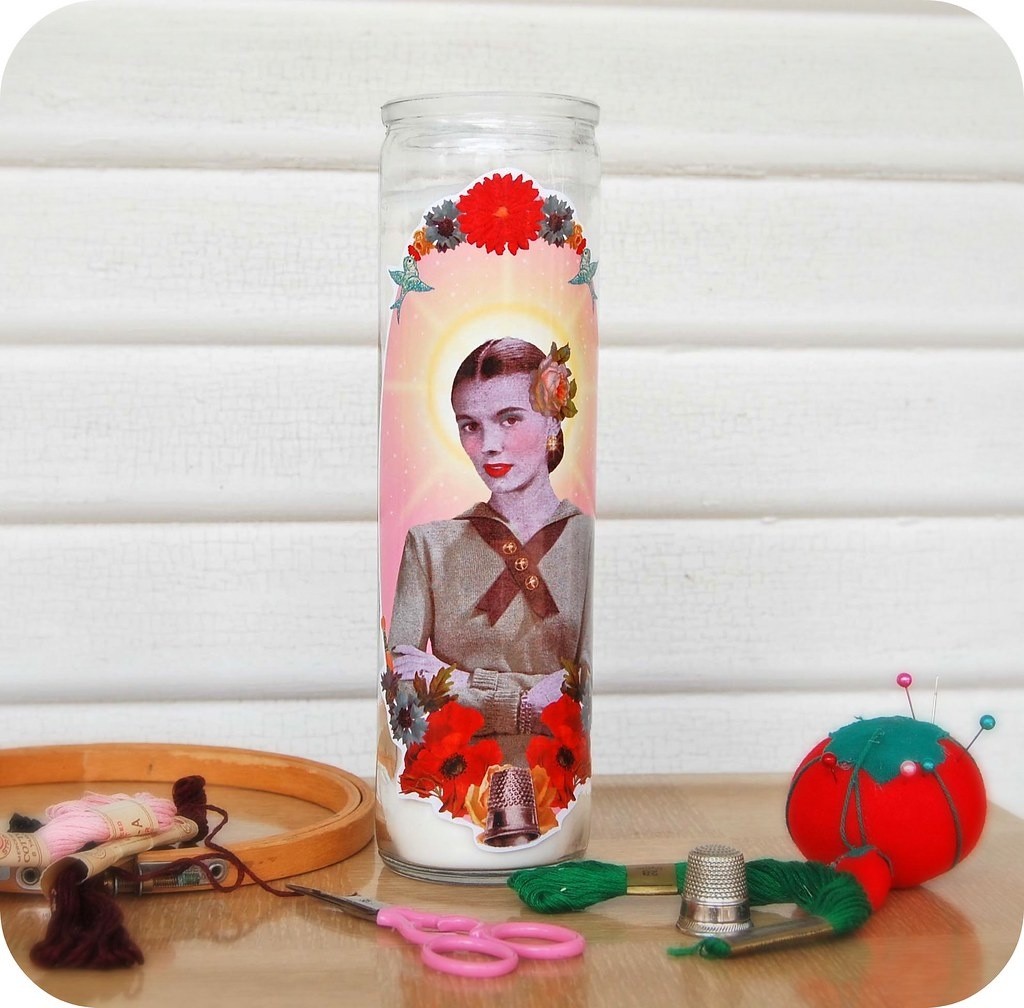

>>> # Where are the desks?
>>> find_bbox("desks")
[0,768,1024,1008]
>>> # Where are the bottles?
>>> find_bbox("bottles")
[367,93,600,884]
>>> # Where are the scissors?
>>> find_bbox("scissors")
[285,883,586,980]
